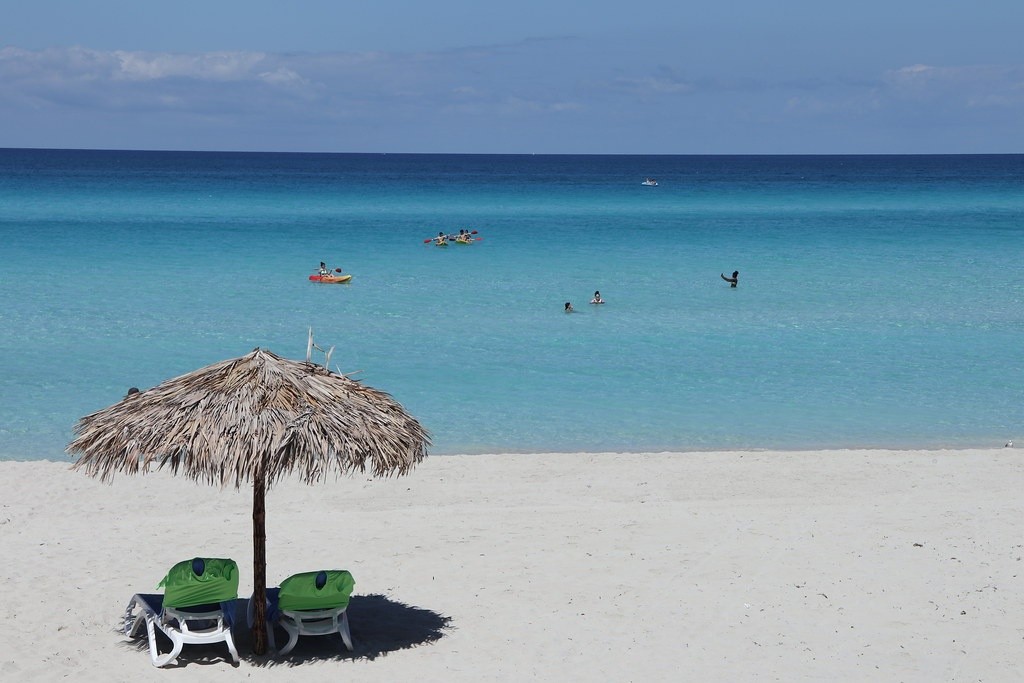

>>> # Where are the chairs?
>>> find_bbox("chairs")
[248,570,355,657]
[125,558,240,669]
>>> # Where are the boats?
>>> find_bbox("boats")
[309,270,352,283]
[641,181,659,186]
[436,241,448,247]
[456,238,472,244]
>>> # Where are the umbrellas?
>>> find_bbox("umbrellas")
[64,325,435,653]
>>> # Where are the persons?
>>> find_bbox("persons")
[591,291,605,303]
[433,232,448,243]
[565,302,573,312]
[456,230,471,242]
[650,179,656,184]
[721,271,739,288]
[318,262,334,277]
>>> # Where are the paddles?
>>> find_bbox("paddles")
[314,268,341,273]
[424,230,482,243]
